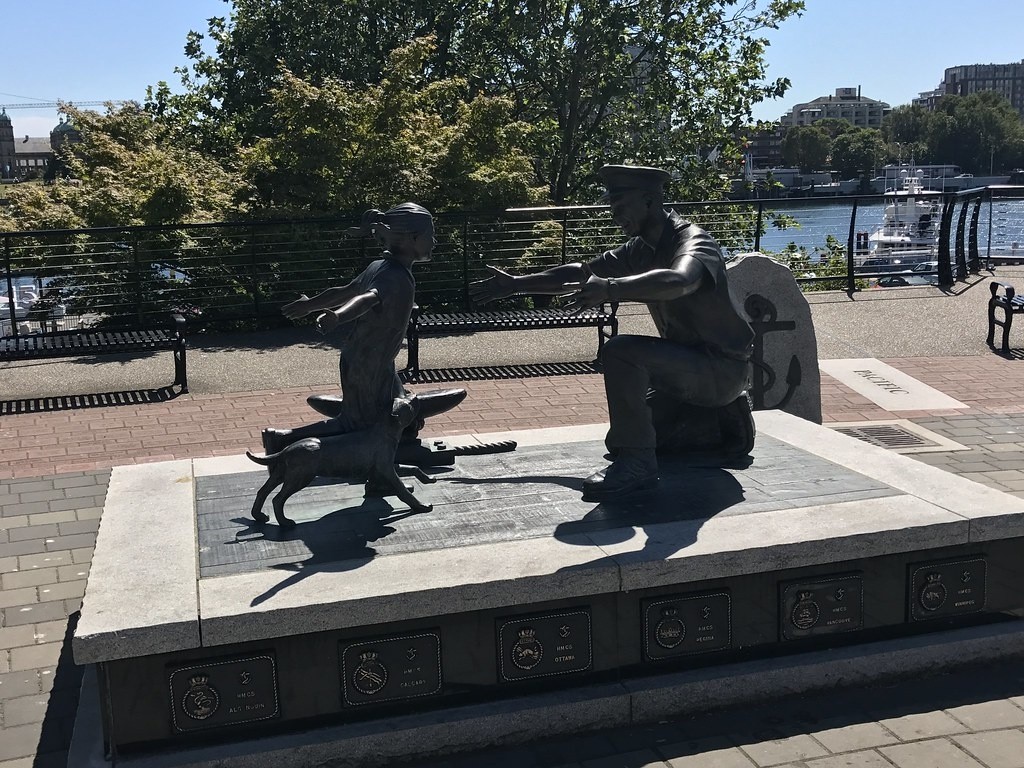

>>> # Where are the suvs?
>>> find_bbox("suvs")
[870,177,886,181]
[954,173,973,178]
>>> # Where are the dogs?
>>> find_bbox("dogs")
[245,393,434,527]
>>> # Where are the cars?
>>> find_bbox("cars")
[1012,169,1024,174]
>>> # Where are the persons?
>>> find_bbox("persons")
[468,165,756,498]
[262,202,437,479]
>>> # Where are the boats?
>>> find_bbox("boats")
[856,141,957,275]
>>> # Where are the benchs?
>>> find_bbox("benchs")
[985,281,1024,351]
[408,293,619,373]
[0,314,189,393]
